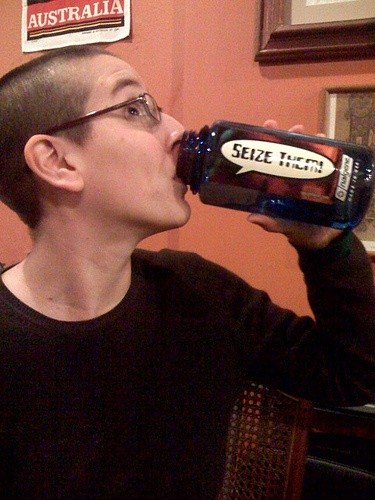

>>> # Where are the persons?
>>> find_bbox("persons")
[0,45,375,500]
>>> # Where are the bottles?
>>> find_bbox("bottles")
[174,120,375,230]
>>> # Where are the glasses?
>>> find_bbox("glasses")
[22,93,162,173]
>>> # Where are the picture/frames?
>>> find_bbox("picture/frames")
[317,83,375,260]
[253,0,375,62]
